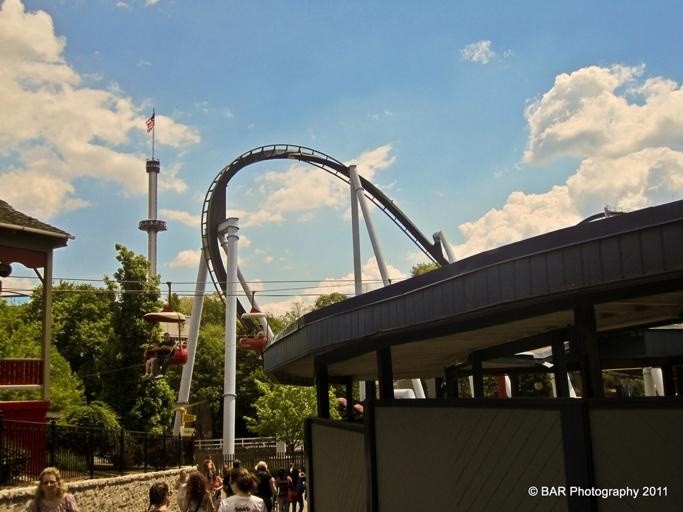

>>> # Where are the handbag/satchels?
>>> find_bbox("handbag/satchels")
[296,479,304,494]
[287,488,299,502]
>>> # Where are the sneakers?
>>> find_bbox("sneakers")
[141,373,165,380]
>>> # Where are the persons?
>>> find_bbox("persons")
[255,324,265,339]
[30,465,78,512]
[153,332,176,379]
[143,339,160,379]
[148,450,307,512]
